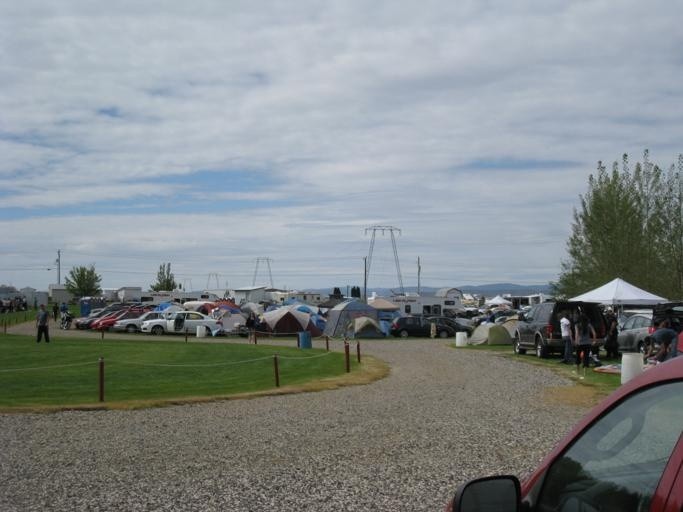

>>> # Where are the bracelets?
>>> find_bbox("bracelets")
[654,355,657,359]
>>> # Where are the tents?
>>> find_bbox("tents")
[322,299,380,337]
[151,297,326,336]
[452,311,529,345]
[339,316,389,338]
[368,298,402,328]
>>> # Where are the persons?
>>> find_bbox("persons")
[35,304,50,344]
[560,310,574,362]
[51,302,59,322]
[0,294,38,313]
[244,310,257,344]
[642,327,680,363]
[571,312,597,380]
[603,306,619,359]
[59,302,68,318]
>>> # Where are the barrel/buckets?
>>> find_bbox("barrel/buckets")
[197,326,205,337]
[298,332,312,349]
[620,353,643,383]
[456,332,467,347]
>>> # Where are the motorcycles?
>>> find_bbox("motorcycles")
[60,311,75,330]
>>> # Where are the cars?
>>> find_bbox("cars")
[74,300,223,336]
[388,310,507,338]
[447,345,683,512]
[514,299,681,358]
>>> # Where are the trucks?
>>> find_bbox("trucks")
[3,300,25,312]
